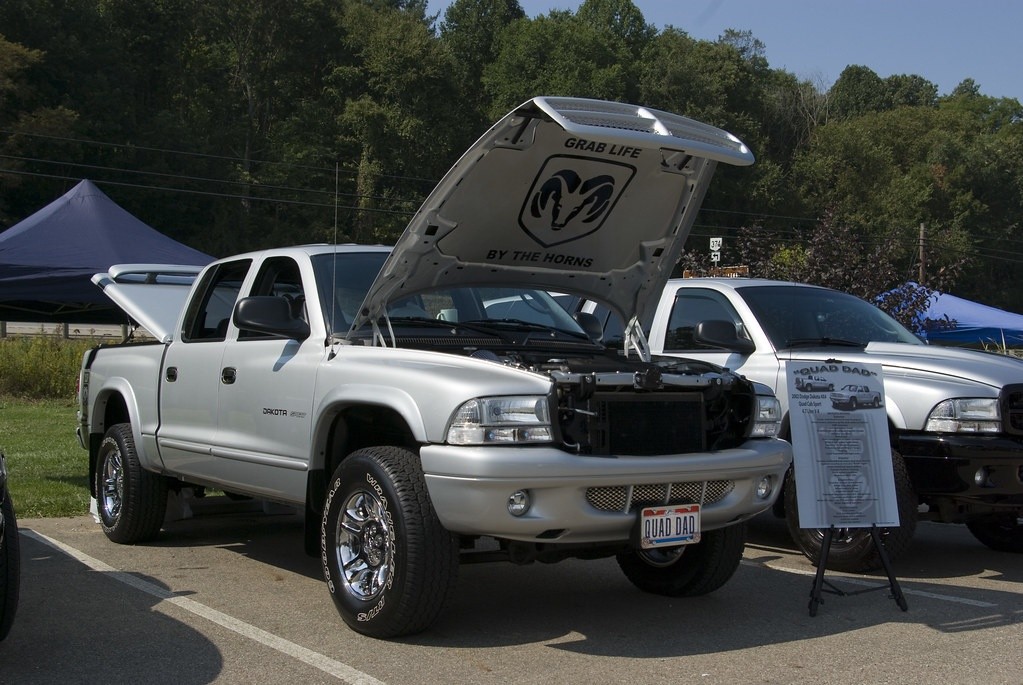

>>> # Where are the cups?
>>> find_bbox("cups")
[437,309,457,323]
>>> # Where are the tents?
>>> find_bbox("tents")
[854,281,1023,358]
[0,179,223,337]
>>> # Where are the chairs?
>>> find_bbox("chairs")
[214,317,229,338]
[289,293,345,326]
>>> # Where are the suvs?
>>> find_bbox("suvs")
[829,384,881,409]
[76,97,792,638]
[435,274,1023,571]
[795,375,835,392]
